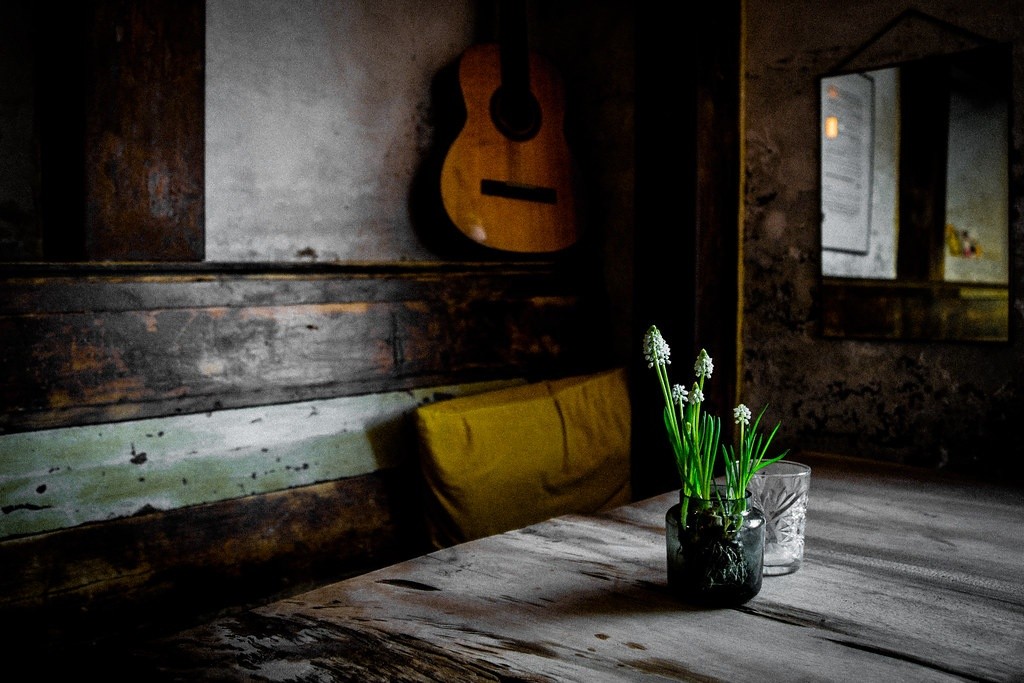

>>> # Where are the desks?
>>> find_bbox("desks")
[159,460,1024,683]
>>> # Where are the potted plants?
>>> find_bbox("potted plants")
[642,325,791,609]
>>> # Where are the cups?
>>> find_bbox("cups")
[725,459,812,576]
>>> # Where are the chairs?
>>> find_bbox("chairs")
[415,367,634,551]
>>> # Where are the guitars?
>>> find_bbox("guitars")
[423,0,578,254]
